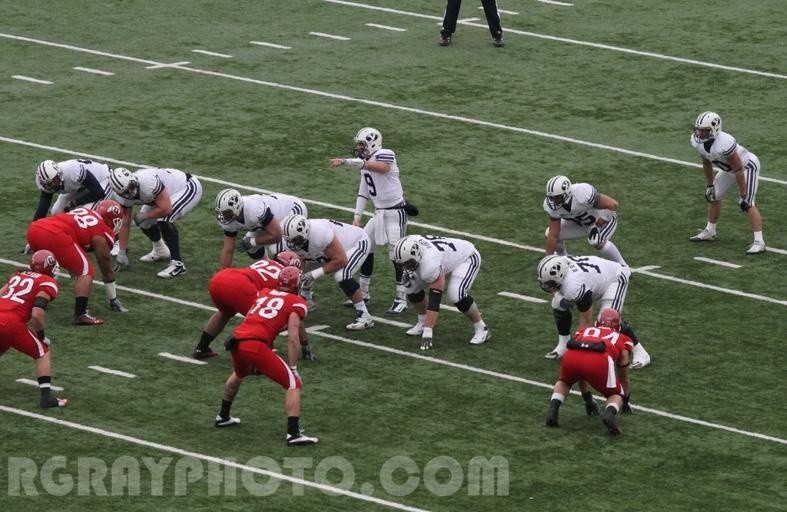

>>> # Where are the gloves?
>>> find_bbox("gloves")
[705,185,716,202]
[110,295,128,312]
[581,391,599,415]
[303,347,316,362]
[621,395,633,415]
[419,327,433,350]
[738,197,749,211]
[37,329,50,345]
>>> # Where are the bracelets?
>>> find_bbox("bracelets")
[103,279,117,301]
[249,237,256,247]
[421,326,433,338]
[310,266,325,280]
[582,391,592,401]
[36,329,45,340]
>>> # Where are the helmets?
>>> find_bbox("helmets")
[546,175,574,210]
[109,168,140,201]
[95,201,125,236]
[693,110,723,144]
[395,237,424,275]
[352,127,383,160]
[215,186,309,293]
[536,255,569,294]
[594,308,620,333]
[36,158,65,194]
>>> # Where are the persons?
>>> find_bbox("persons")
[542,175,633,281]
[328,126,408,314]
[536,254,651,370]
[281,214,375,330]
[214,265,319,446]
[191,251,318,363]
[26,199,129,325]
[21,156,115,256]
[689,111,766,254]
[438,0,505,47]
[546,308,632,435]
[109,167,203,279]
[395,234,492,351]
[0,249,69,408]
[214,188,309,271]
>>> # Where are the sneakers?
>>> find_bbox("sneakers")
[628,352,651,370]
[547,408,558,426]
[602,413,623,435]
[490,36,505,48]
[214,413,242,426]
[620,263,632,280]
[546,345,568,359]
[689,228,717,241]
[286,430,319,447]
[748,240,765,254]
[193,348,219,359]
[109,237,185,278]
[72,309,106,325]
[299,291,491,346]
[41,396,69,408]
[438,34,452,46]
